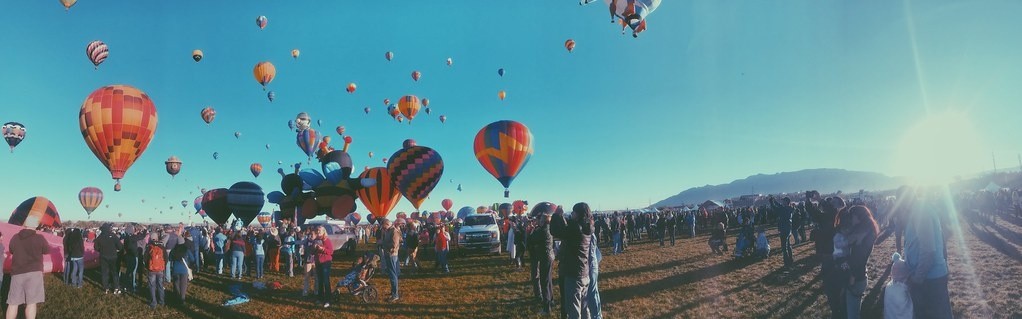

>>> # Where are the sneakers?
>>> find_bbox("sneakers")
[103,289,109,295]
[121,287,127,293]
[388,296,400,303]
[113,288,122,295]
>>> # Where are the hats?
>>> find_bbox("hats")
[150,233,159,242]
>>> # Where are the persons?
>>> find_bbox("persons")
[143,232,167,308]
[524,214,556,314]
[0,197,1021,270]
[805,190,845,319]
[67,229,85,287]
[63,229,72,283]
[884,253,914,319]
[121,226,145,292]
[230,231,245,280]
[550,202,605,319]
[309,226,333,308]
[213,226,228,274]
[5,216,50,319]
[895,185,953,319]
[281,230,320,298]
[378,219,400,302]
[169,236,195,303]
[94,223,123,294]
[1,232,4,289]
[833,205,879,319]
[267,227,279,275]
[254,234,266,279]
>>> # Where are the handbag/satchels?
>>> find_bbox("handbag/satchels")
[187,267,194,282]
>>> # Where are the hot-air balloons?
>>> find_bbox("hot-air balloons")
[565,39,575,53]
[3,0,558,235]
[605,0,663,38]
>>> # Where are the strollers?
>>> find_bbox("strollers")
[334,252,382,303]
[733,224,755,259]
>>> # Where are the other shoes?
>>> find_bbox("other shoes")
[514,267,526,272]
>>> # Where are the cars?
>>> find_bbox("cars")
[458,213,501,256]
[298,223,357,252]
